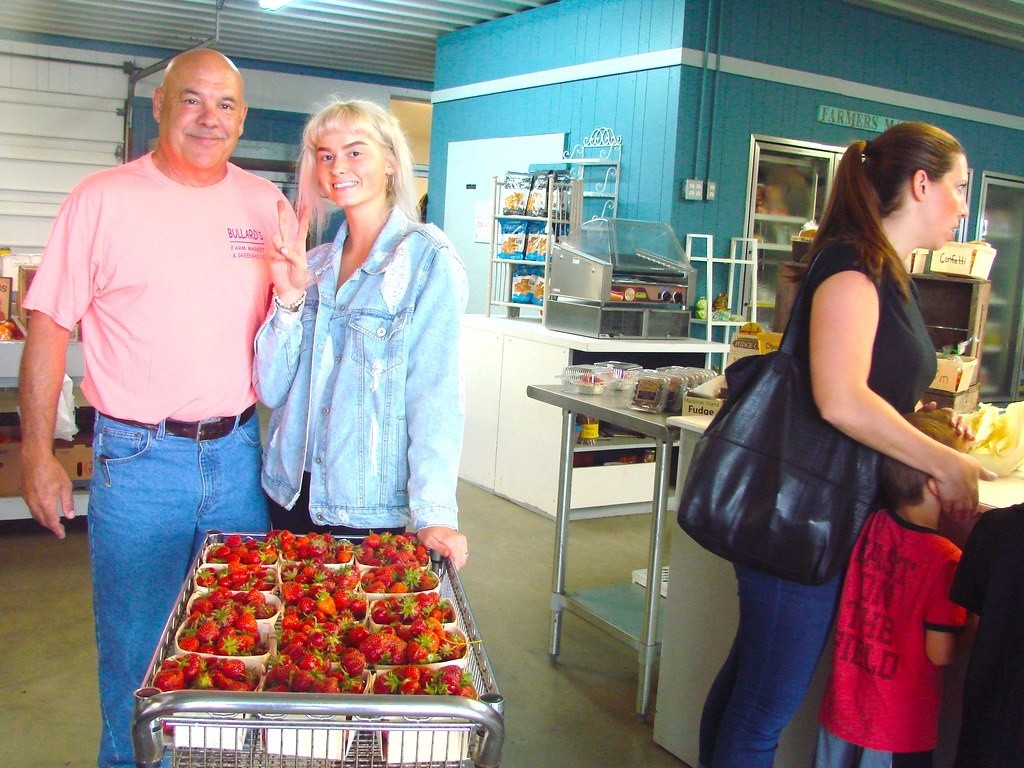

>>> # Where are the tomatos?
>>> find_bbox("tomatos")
[0,322,21,341]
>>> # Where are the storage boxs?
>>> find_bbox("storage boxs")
[152,540,479,763]
[682,386,728,418]
[930,351,976,393]
[55,434,93,494]
[0,426,26,497]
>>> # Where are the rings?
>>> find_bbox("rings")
[462,553,469,557]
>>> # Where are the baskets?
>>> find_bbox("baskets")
[150,540,473,764]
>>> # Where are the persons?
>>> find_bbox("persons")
[947,502,1024,768]
[814,413,968,768]
[696,122,998,768]
[19,49,299,768]
[253,99,471,571]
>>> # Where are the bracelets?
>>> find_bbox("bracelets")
[272,288,307,309]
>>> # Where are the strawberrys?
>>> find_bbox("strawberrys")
[154,530,473,750]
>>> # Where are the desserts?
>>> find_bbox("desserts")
[573,374,604,395]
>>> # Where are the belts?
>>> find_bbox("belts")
[99,401,258,443]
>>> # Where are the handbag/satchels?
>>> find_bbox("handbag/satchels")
[678,241,880,586]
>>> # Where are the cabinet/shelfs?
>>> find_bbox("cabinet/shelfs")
[489,173,586,324]
